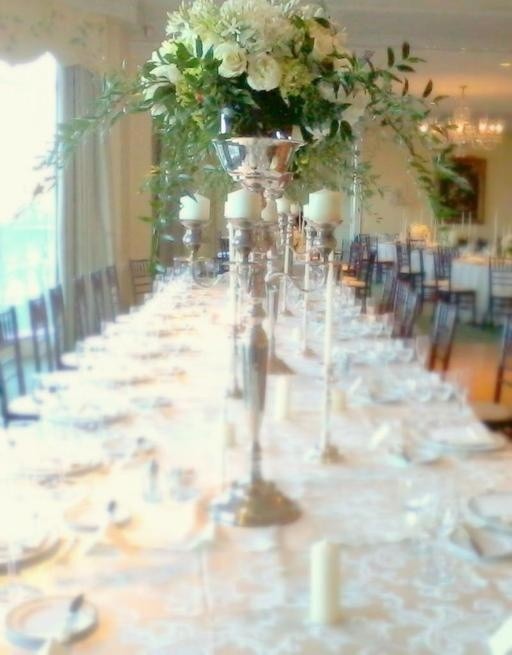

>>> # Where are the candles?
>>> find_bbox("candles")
[175,182,346,229]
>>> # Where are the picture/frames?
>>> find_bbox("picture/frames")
[433,154,487,225]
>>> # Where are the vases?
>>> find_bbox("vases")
[214,135,300,188]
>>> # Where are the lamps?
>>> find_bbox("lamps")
[415,84,504,150]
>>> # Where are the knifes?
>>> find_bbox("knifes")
[57,591,89,645]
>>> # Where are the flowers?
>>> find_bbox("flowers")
[19,0,474,281]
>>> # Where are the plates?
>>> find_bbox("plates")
[7,596,98,642]
[2,275,512,629]
